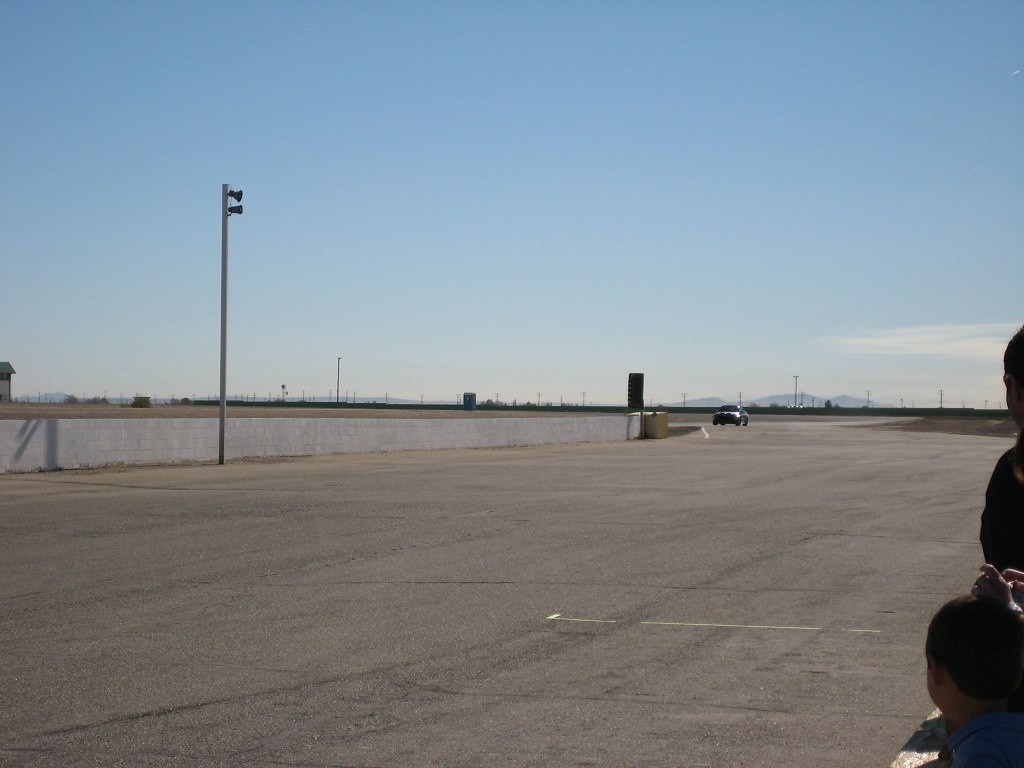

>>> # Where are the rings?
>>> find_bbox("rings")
[973,584,977,590]
[984,576,989,582]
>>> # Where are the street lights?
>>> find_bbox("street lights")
[336,357,341,402]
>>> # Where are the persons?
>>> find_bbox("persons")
[887,323,1024,768]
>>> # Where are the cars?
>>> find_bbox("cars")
[713,405,749,426]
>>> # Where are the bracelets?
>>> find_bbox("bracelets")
[1011,605,1024,613]
